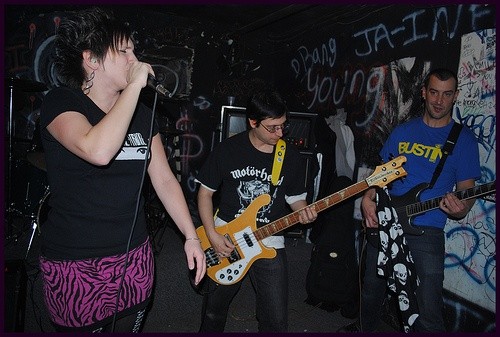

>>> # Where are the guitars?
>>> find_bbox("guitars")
[360,180,496,252]
[194,153,409,286]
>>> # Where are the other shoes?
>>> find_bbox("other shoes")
[338,322,377,333]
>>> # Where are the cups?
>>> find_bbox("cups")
[227,96,236,106]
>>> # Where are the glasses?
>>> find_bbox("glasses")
[256,120,291,134]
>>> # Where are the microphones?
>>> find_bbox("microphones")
[146,73,172,98]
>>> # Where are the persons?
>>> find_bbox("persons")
[337,69,479,332]
[26,9,206,332]
[192,84,318,331]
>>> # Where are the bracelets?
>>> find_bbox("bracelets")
[186,238,199,240]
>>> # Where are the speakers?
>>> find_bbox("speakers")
[3,257,27,332]
[283,153,312,236]
